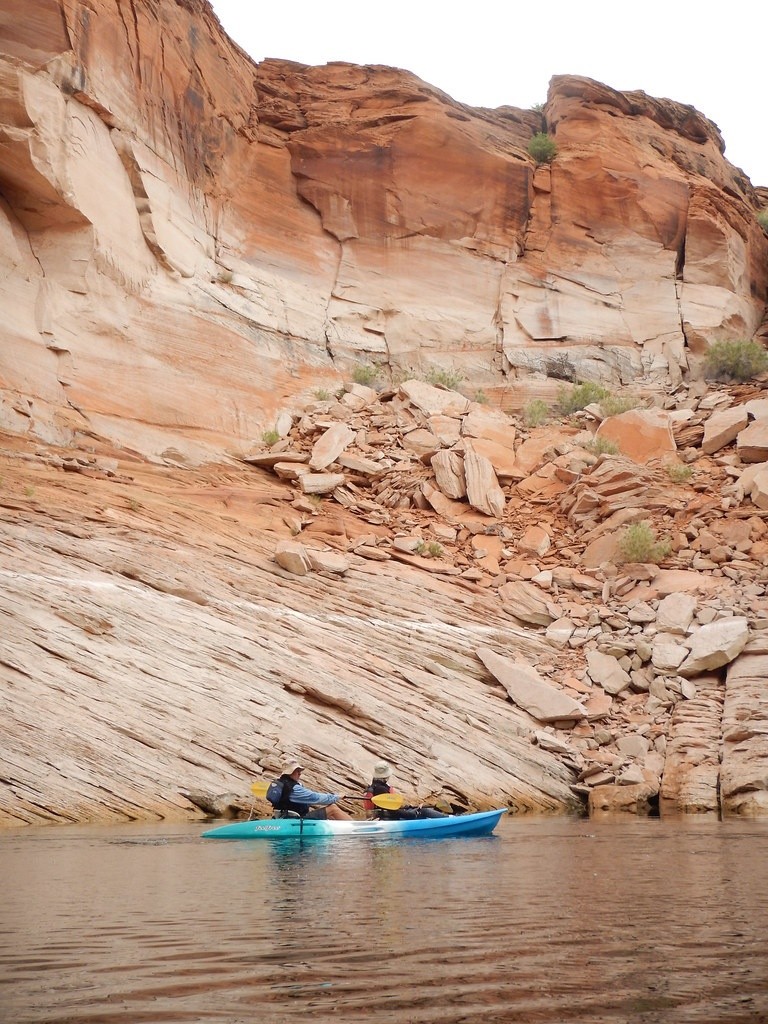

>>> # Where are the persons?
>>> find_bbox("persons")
[272,758,380,822]
[365,761,464,820]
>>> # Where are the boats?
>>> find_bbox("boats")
[200,808,508,839]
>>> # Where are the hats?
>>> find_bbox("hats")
[372,761,396,779]
[279,758,305,778]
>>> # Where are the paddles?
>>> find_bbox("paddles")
[400,799,454,813]
[250,781,404,811]
